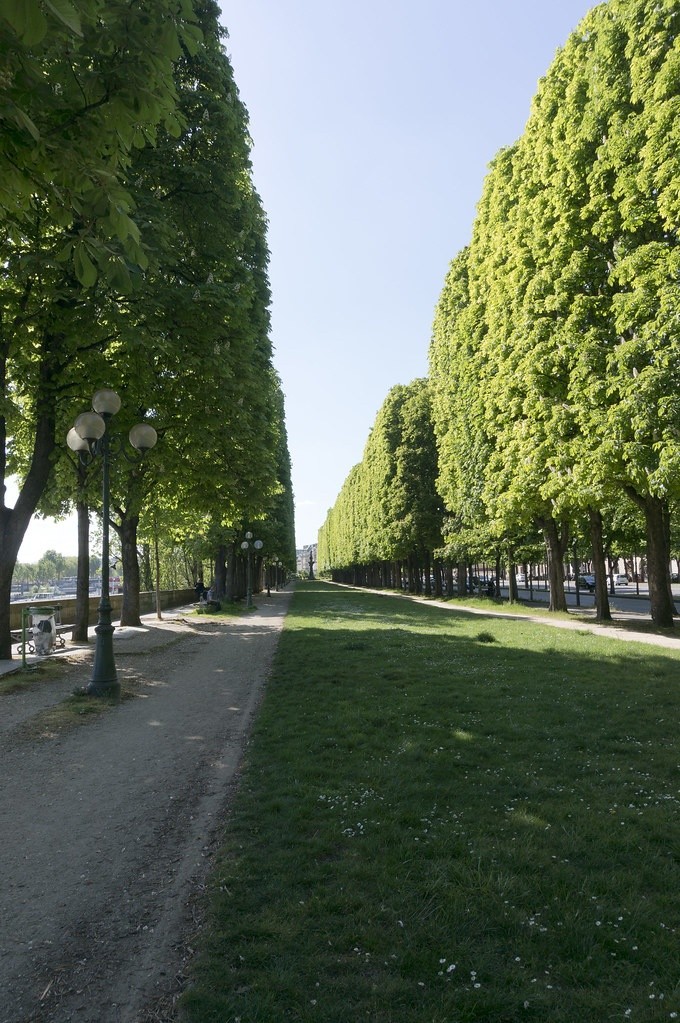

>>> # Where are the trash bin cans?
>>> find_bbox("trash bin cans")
[29,606,57,656]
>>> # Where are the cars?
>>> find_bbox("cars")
[575,570,596,593]
[516,573,526,581]
[606,574,629,587]
[475,573,490,588]
[422,575,434,585]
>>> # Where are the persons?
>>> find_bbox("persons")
[202,588,208,602]
[207,585,216,600]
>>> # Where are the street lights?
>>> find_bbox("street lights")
[272,556,282,592]
[239,531,265,608]
[65,387,157,702]
